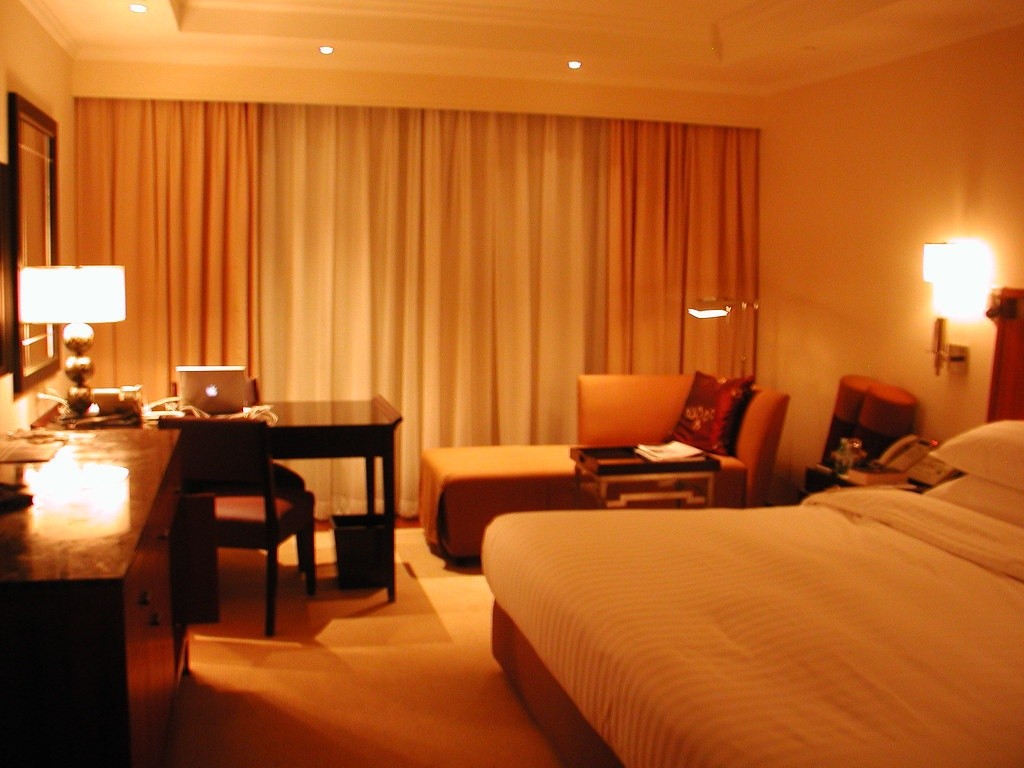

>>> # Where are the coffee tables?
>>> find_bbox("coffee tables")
[575,461,715,513]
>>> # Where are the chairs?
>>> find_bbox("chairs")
[158,414,315,637]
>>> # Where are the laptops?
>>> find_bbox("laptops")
[175,365,245,415]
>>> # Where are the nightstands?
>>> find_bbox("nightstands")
[803,464,926,496]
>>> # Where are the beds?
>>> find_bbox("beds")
[480,288,1024,768]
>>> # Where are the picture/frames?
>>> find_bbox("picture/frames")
[9,91,61,393]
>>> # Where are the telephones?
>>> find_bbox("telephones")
[877,435,938,472]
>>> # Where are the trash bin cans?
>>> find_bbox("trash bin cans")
[329,512,390,589]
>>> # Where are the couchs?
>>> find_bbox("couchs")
[418,369,792,566]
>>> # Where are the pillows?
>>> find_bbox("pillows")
[926,419,1024,492]
[663,368,756,457]
[922,473,1024,527]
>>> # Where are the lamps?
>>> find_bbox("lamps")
[17,263,126,419]
[923,243,975,377]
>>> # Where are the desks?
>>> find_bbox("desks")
[30,394,404,603]
[0,428,182,768]
